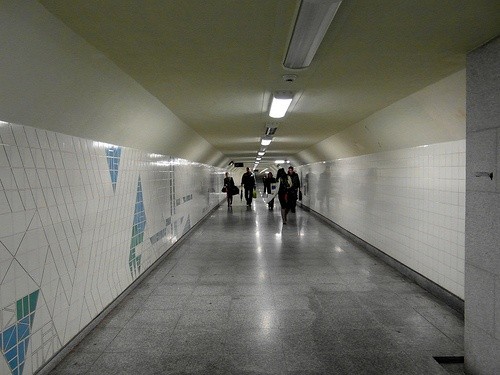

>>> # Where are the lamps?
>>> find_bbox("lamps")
[281,0,344,72]
[268,91,293,119]
[253,122,278,171]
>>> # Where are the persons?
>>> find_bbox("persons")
[263,176,267,193]
[241,167,256,207]
[287,166,300,213]
[224,172,234,207]
[265,172,276,211]
[276,168,293,225]
